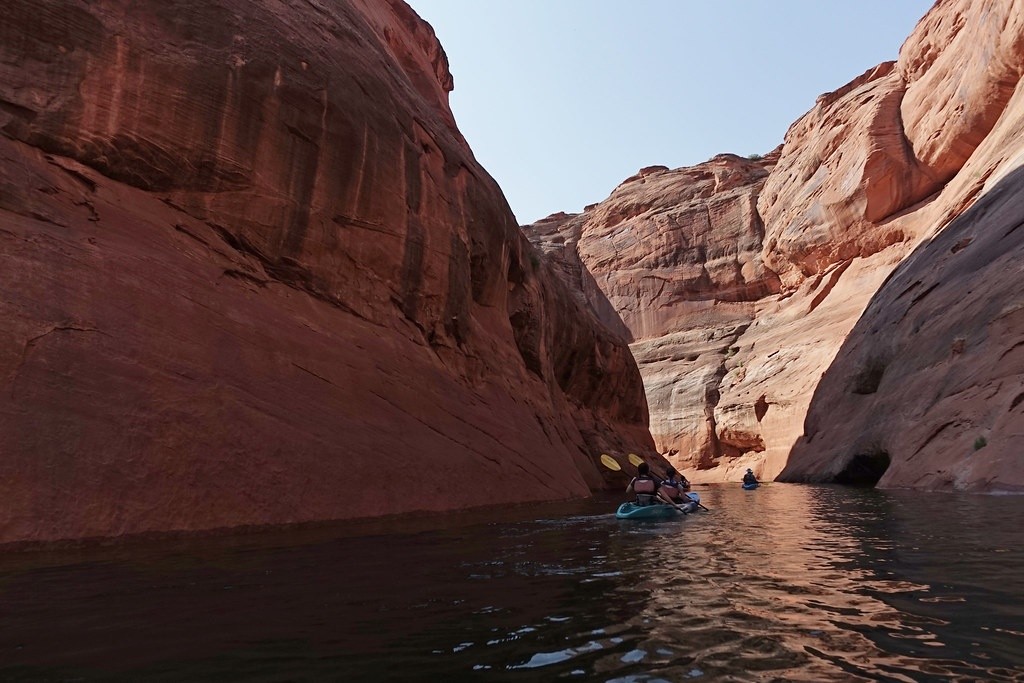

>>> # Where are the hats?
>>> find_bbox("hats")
[746,468,752,472]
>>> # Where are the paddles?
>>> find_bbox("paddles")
[734,480,775,486]
[621,449,711,510]
[598,453,687,515]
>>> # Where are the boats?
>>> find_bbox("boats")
[743,482,759,490]
[616,492,700,518]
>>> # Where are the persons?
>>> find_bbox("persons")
[751,472,758,482]
[680,475,689,488]
[626,463,675,506]
[661,467,690,502]
[744,469,758,484]
